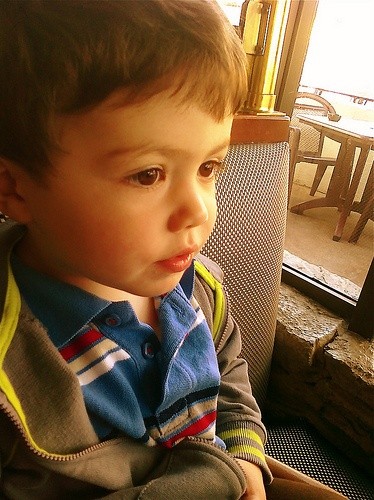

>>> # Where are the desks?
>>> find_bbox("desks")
[289,112,374,242]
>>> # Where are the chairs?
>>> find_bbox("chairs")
[287,92,341,209]
[200,113,374,500]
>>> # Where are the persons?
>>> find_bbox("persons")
[0,0,273,500]
